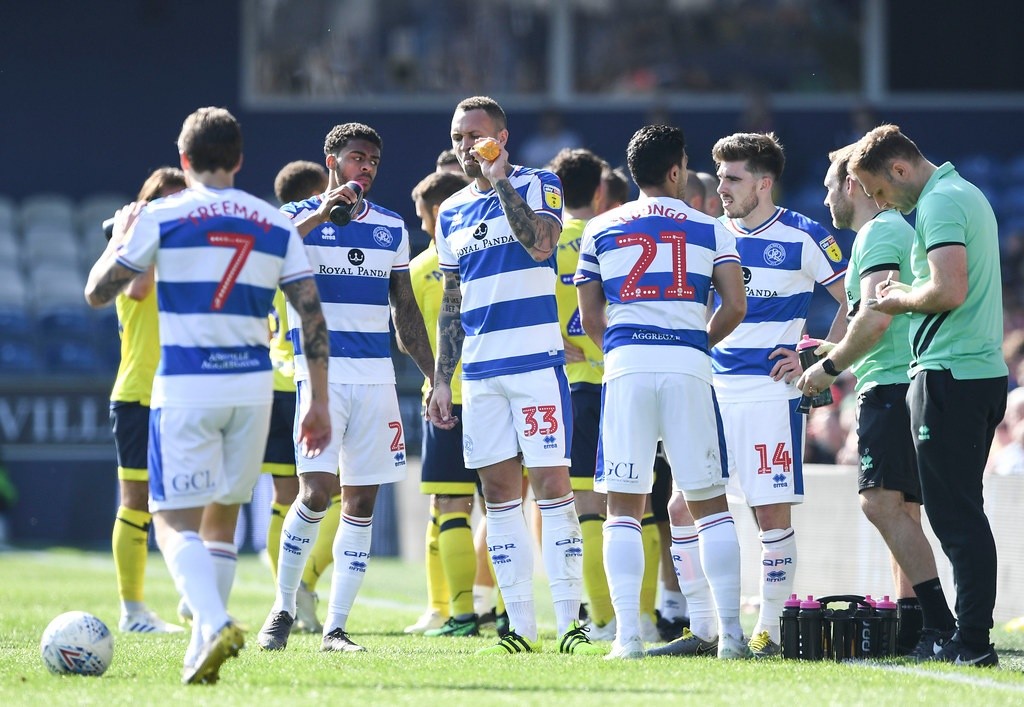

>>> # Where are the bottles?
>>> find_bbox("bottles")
[780,594,801,660]
[102,217,114,242]
[800,596,823,661]
[797,335,833,409]
[469,140,500,165]
[330,180,364,226]
[876,596,897,659]
[857,595,876,659]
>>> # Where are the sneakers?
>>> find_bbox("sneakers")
[581,616,616,641]
[320,627,367,654]
[476,607,498,631]
[747,630,782,662]
[556,618,605,658]
[177,601,249,634]
[119,609,188,638]
[717,632,760,662]
[653,608,690,643]
[256,602,298,655]
[475,628,543,657]
[423,613,479,638]
[641,613,661,641]
[602,633,645,661]
[492,607,510,637]
[921,628,1001,670]
[295,581,324,635]
[645,627,719,659]
[405,609,450,634]
[903,618,959,665]
[181,620,246,685]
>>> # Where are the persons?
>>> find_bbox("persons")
[390,94,748,660]
[846,124,1012,670]
[255,121,434,655]
[260,159,345,639]
[84,105,332,684]
[794,137,957,664]
[643,131,849,657]
[104,166,187,638]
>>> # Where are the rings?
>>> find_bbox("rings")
[808,386,814,394]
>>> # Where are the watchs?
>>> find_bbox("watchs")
[822,356,844,377]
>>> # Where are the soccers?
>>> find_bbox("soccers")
[39,610,116,677]
